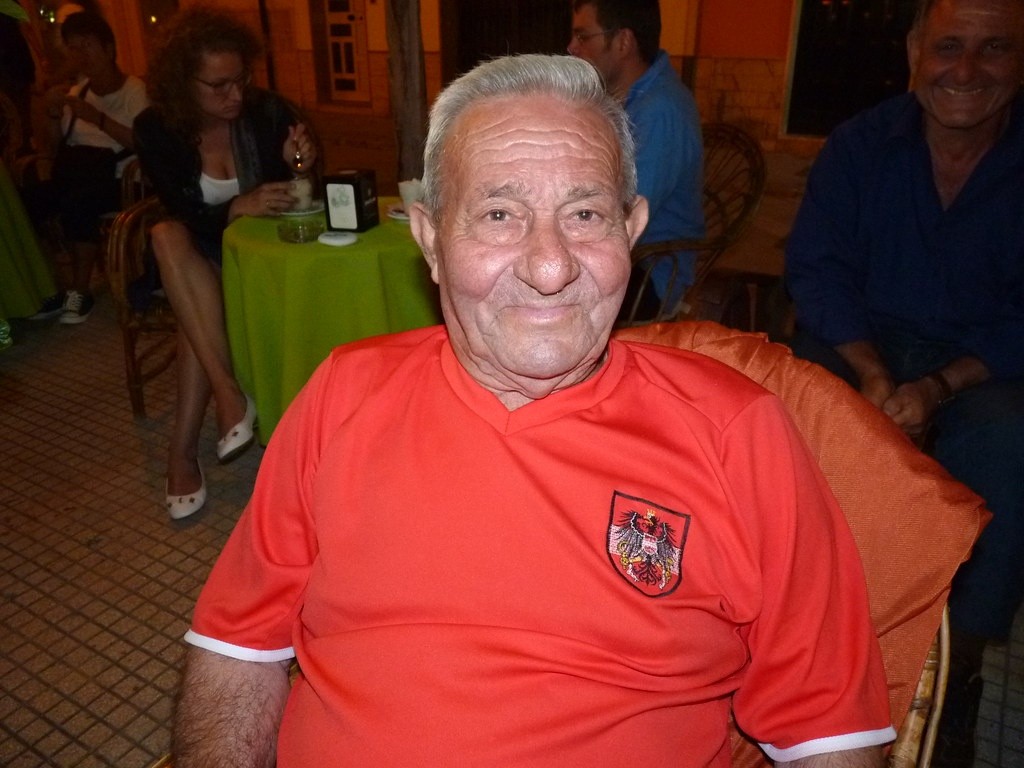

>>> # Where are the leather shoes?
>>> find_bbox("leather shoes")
[163,454,208,520]
[215,393,258,464]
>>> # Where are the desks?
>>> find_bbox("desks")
[221,198,443,438]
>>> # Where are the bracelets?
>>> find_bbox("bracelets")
[100,113,106,129]
[924,371,957,406]
[44,81,48,90]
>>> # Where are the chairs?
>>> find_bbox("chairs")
[105,195,179,415]
[607,317,993,768]
[612,120,763,328]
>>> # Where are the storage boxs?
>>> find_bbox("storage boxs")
[324,168,379,233]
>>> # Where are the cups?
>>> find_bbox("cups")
[398,181,419,216]
[290,174,314,209]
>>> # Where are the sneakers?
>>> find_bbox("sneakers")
[58,288,97,323]
[27,296,65,321]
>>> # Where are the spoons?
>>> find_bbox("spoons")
[294,141,303,166]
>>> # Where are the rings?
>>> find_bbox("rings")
[266,200,271,206]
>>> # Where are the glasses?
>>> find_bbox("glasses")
[572,29,615,47]
[195,64,254,97]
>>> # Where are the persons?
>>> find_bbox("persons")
[568,0,702,317]
[791,0,1024,768]
[134,17,313,520]
[169,56,897,768]
[43,10,146,327]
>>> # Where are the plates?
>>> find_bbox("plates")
[387,213,408,219]
[280,200,324,215]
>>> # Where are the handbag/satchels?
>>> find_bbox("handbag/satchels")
[36,141,74,200]
